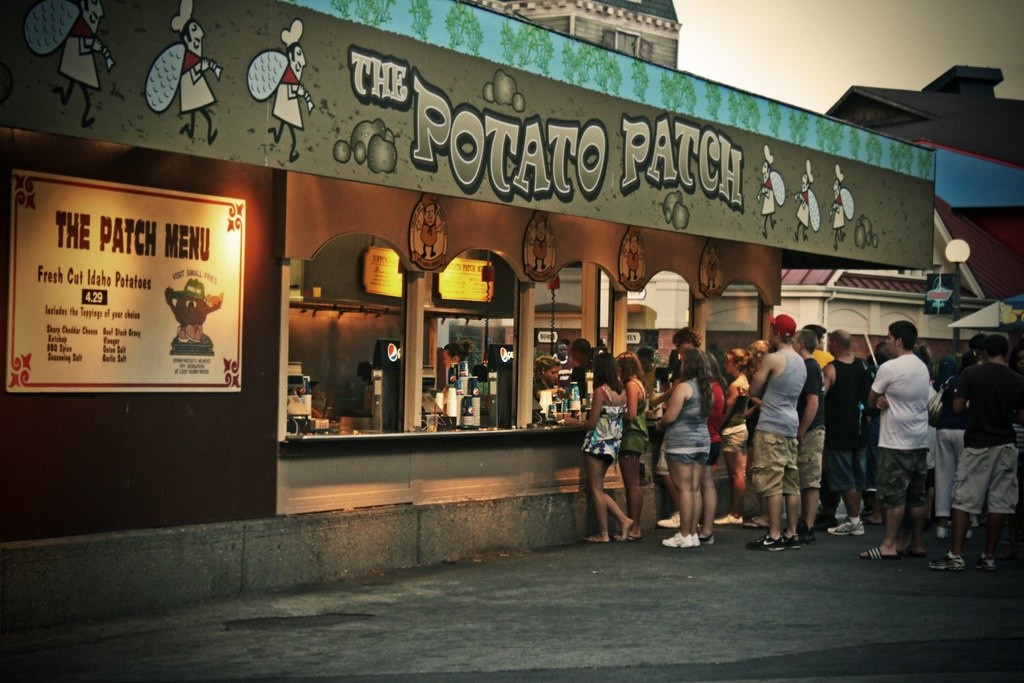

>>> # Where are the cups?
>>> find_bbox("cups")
[434,361,481,427]
[549,385,582,426]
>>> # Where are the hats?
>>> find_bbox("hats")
[769,315,796,337]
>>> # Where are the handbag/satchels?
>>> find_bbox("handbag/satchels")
[927,374,955,427]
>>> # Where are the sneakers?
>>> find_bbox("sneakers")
[747,531,785,550]
[662,532,694,548]
[827,518,864,535]
[782,528,800,550]
[928,550,966,570]
[693,533,700,547]
[974,554,997,571]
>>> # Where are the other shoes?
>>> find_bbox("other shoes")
[814,513,838,531]
[699,535,714,544]
[656,513,700,528]
[744,512,769,529]
[996,549,1019,561]
[713,514,744,525]
[797,521,816,546]
[862,509,980,540]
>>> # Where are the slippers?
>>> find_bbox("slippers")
[614,533,642,542]
[860,547,899,560]
[900,545,925,558]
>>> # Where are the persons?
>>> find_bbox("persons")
[533,314,1024,572]
[441,338,472,376]
